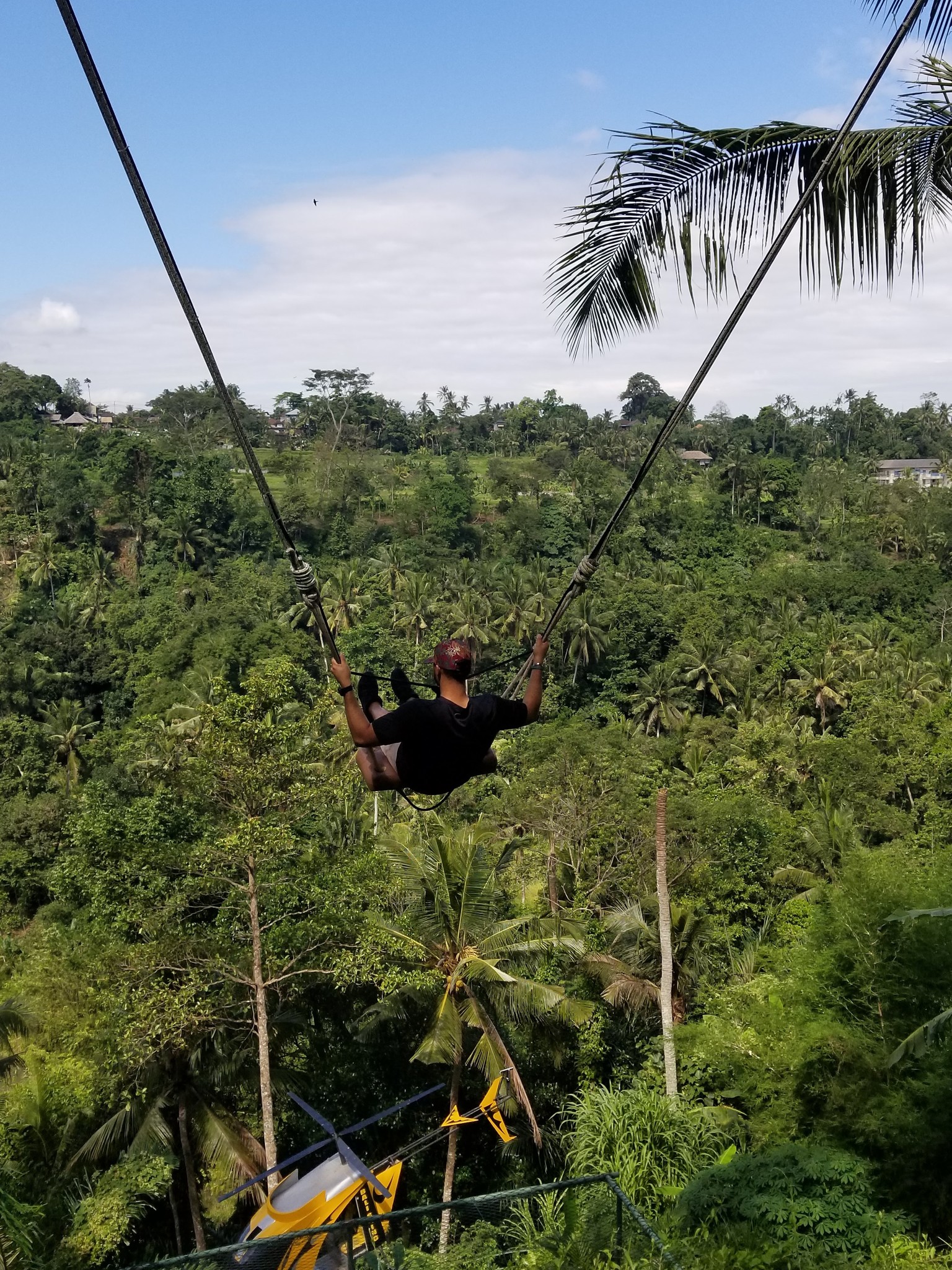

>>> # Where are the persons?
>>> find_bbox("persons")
[331,634,550,796]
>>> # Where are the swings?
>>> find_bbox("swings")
[58,0,929,793]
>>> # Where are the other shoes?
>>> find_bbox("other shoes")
[357,671,383,723]
[391,667,420,703]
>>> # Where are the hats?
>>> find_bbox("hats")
[423,639,472,670]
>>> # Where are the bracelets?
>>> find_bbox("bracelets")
[530,662,544,670]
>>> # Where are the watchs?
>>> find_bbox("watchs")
[338,684,353,697]
